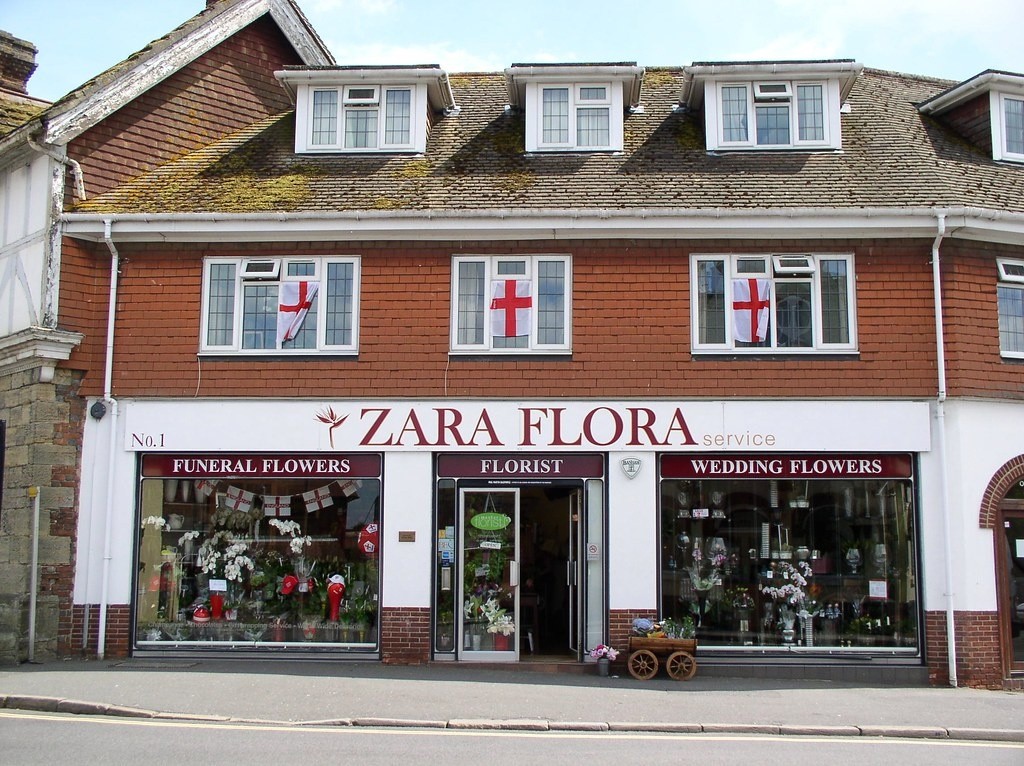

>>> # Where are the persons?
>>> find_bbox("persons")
[525,549,556,651]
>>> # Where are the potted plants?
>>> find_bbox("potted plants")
[849,616,915,647]
[176,557,374,643]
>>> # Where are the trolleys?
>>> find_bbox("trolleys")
[611,635,698,681]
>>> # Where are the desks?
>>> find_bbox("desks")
[506,595,540,654]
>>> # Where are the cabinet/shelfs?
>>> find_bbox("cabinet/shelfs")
[161,501,210,555]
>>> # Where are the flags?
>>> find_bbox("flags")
[337,479,362,497]
[731,279,770,342]
[277,282,319,342]
[264,496,290,516]
[303,486,333,513]
[490,281,533,337]
[225,486,253,513]
[194,478,219,496]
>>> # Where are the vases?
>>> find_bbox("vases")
[495,633,509,651]
[846,549,862,574]
[341,622,350,643]
[439,636,451,648]
[464,633,470,649]
[357,621,366,642]
[597,658,610,676]
[821,617,838,634]
[782,629,795,643]
[695,589,708,626]
[162,479,207,503]
[869,544,892,574]
[472,634,482,650]
[810,556,830,574]
[792,546,811,561]
[732,605,751,620]
[810,618,813,647]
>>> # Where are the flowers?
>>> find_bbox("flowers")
[434,530,516,636]
[137,500,312,583]
[631,616,695,640]
[589,645,620,661]
[664,542,839,632]
